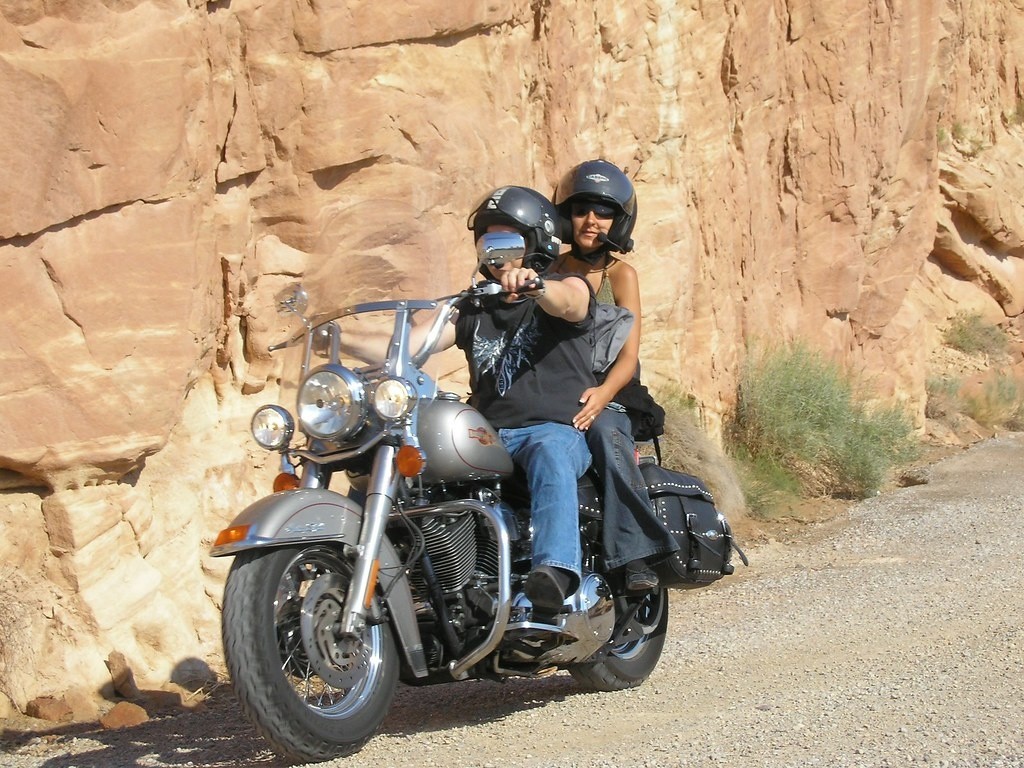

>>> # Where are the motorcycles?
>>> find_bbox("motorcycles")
[204,232,672,763]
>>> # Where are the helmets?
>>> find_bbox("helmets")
[467,186,562,281]
[551,158,638,252]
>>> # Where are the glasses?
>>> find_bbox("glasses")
[567,193,622,219]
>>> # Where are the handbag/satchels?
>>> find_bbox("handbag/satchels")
[595,359,666,441]
[639,463,749,589]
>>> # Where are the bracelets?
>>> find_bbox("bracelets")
[522,276,546,300]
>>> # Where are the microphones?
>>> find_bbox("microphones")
[495,253,543,269]
[598,232,626,254]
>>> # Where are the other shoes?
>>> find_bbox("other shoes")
[524,564,572,626]
[625,558,660,591]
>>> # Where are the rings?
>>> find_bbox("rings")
[591,415,595,421]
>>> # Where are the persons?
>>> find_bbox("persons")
[313,161,665,625]
